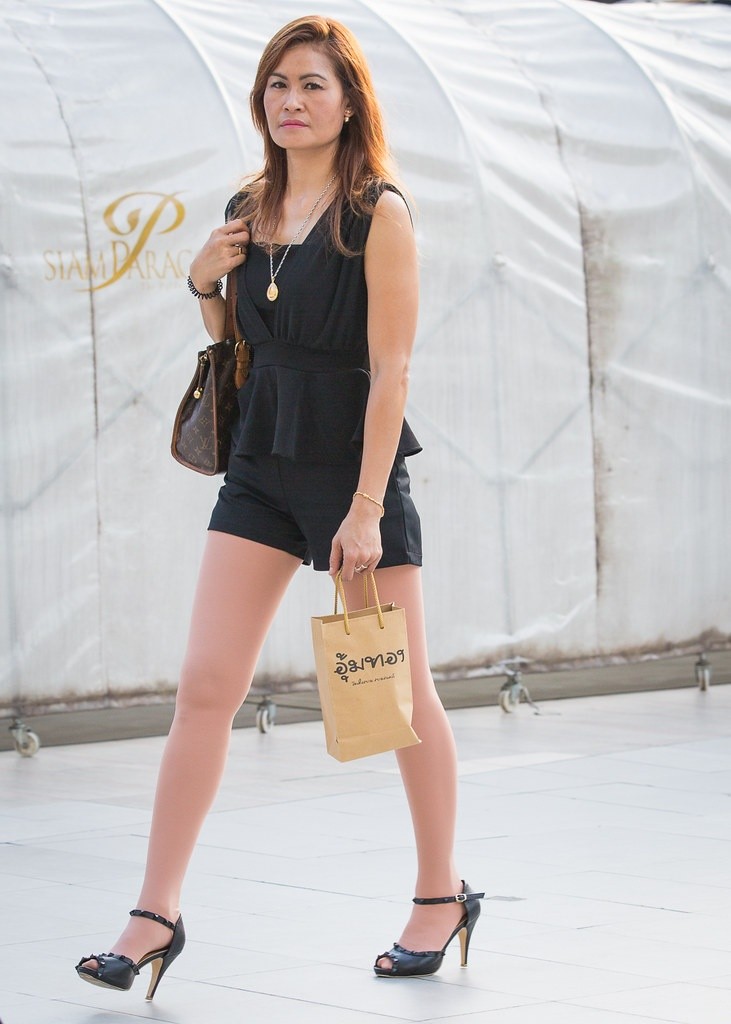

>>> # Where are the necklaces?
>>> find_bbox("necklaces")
[267,174,336,301]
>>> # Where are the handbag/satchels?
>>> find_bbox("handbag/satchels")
[313,562,421,760]
[171,264,246,476]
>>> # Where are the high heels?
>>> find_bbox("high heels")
[374,882,485,978]
[75,907,187,1002]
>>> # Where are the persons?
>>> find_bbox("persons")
[76,15,485,1001]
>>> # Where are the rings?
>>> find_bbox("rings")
[238,246,242,255]
[355,565,367,572]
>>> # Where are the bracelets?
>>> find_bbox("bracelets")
[353,492,384,518]
[187,275,223,299]
